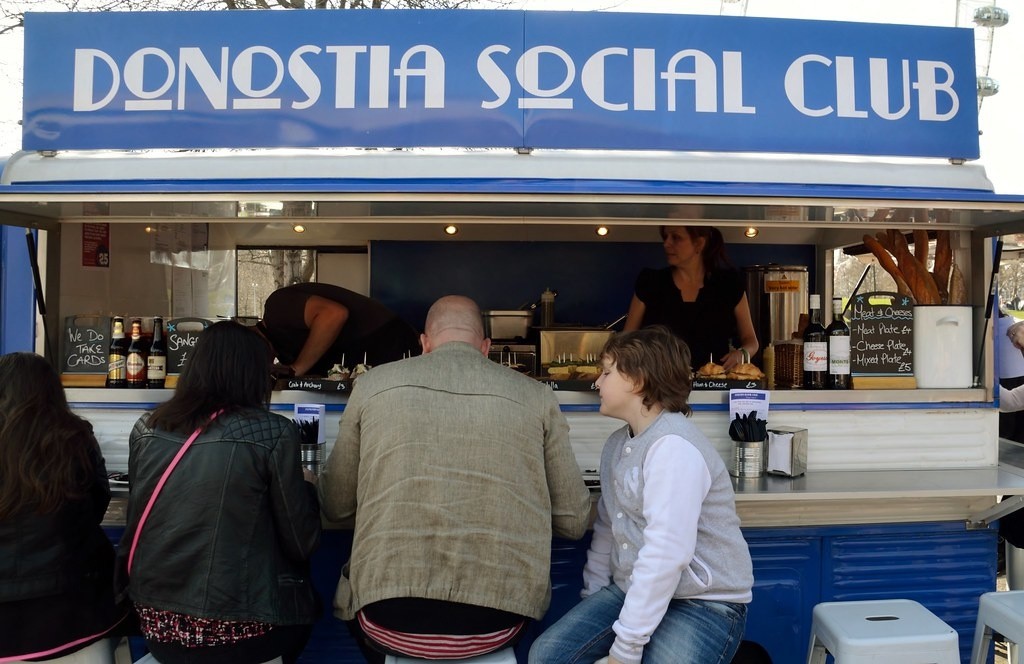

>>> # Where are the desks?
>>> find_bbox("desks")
[97,437,1024,532]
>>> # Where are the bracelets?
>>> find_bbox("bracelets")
[739,346,750,363]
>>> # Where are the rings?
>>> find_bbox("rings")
[1011,332,1015,336]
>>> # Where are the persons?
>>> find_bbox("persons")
[528,326,754,664]
[240,282,422,383]
[317,295,591,664]
[617,225,760,373]
[117,322,326,664]
[1,353,146,664]
[999,305,1024,591]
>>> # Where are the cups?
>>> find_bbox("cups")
[730,438,764,478]
[301,441,326,482]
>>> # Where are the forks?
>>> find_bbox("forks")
[735,420,745,441]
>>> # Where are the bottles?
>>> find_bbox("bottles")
[826,297,850,390]
[541,288,554,327]
[126,320,147,389]
[803,294,828,390]
[146,319,167,389]
[108,316,127,388]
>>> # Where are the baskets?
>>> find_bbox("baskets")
[774,337,804,387]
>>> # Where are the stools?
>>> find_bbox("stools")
[42,588,1024,664]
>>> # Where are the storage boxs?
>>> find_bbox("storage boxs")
[772,337,804,389]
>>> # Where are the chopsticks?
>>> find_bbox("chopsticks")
[290,416,319,444]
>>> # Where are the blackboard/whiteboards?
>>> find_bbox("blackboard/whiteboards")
[64,314,110,373]
[167,317,213,374]
[850,292,914,376]
